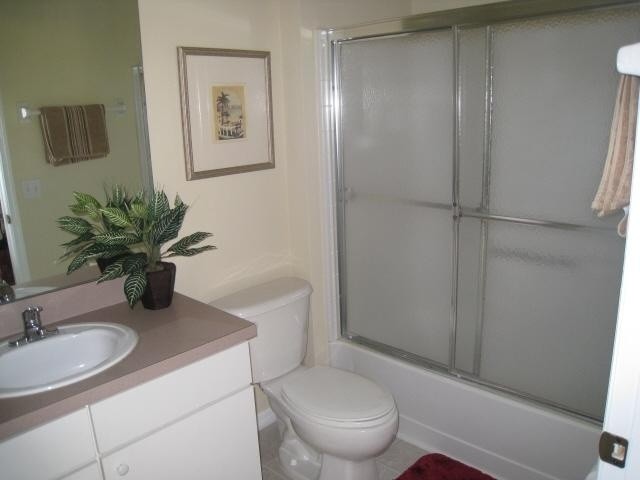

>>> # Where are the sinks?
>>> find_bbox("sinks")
[0,322,138,399]
[10,286,60,300]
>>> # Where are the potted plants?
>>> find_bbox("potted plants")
[81,189,218,310]
[50,181,152,277]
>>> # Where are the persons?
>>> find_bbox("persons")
[0,214,16,286]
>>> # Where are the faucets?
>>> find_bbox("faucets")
[0,278,15,304]
[22,306,46,340]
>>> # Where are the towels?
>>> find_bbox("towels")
[590,75,639,238]
[38,104,112,167]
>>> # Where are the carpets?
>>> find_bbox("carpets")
[394,453,498,480]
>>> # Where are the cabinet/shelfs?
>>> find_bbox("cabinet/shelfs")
[1,340,264,480]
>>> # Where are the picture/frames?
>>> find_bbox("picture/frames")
[177,45,277,182]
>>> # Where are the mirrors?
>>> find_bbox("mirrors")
[0,0,161,306]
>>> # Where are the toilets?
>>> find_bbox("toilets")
[209,276,397,479]
